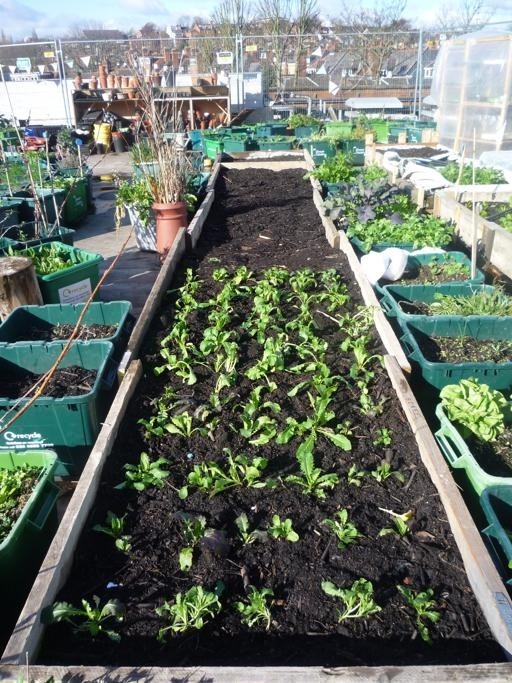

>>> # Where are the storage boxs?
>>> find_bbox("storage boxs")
[189,130,201,151]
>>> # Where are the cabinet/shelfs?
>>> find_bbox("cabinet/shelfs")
[73,86,230,152]
[436,34,512,161]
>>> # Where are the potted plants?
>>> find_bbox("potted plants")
[201,113,365,166]
[112,170,198,251]
[0,119,104,305]
[309,159,512,315]
[0,449,62,568]
[132,140,171,179]
[399,316,512,420]
[434,374,512,501]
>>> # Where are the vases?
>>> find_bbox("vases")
[110,132,125,152]
[0,301,132,359]
[479,484,512,562]
[128,91,136,99]
[150,200,189,256]
[101,93,111,101]
[115,92,127,99]
[0,341,115,448]
[369,121,436,144]
